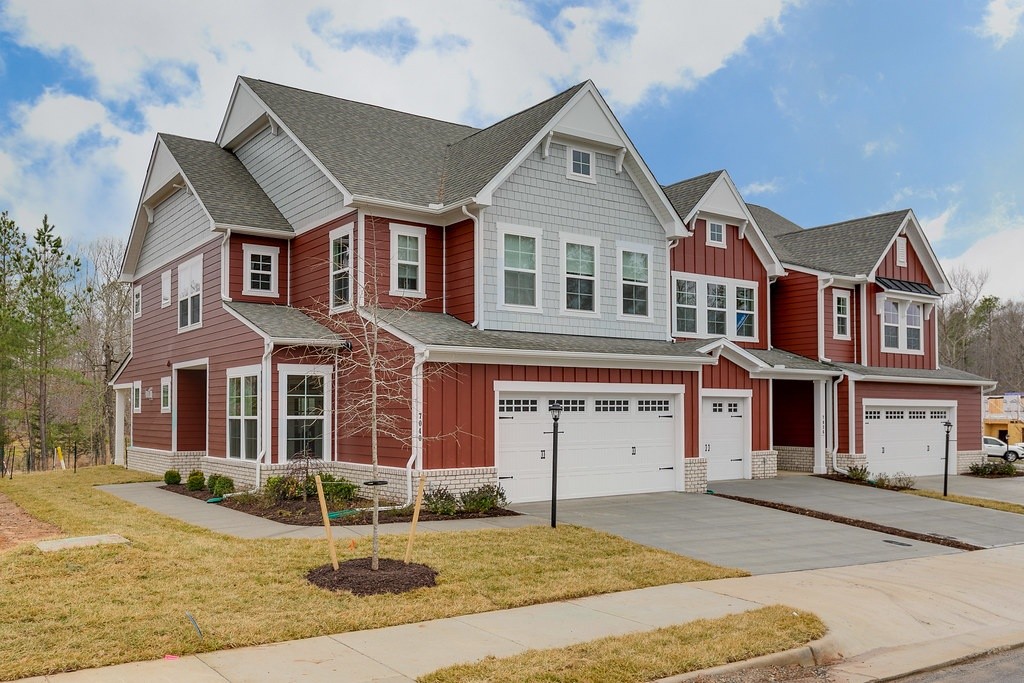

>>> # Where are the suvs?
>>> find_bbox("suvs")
[983,436,1024,462]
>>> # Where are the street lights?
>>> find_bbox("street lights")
[943,422,953,496]
[549,404,562,528]
[1005,434,1010,451]
